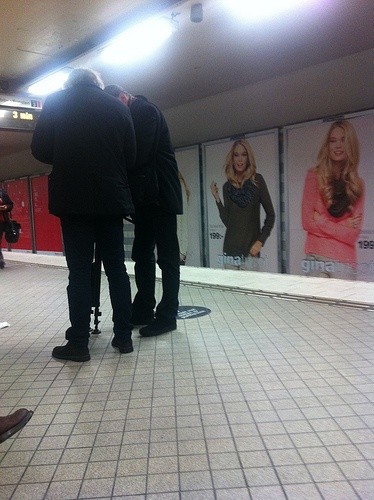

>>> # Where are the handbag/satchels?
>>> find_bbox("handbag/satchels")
[2,202,20,243]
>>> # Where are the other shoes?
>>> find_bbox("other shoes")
[139,318,178,337]
[52,341,91,363]
[130,313,154,325]
[111,336,134,354]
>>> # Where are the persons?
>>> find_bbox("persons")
[105,84,182,337]
[210,141,275,269]
[31,68,134,363]
[302,120,364,278]
[176,168,189,265]
[0,187,35,442]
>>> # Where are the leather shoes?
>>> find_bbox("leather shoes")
[0,408,35,444]
